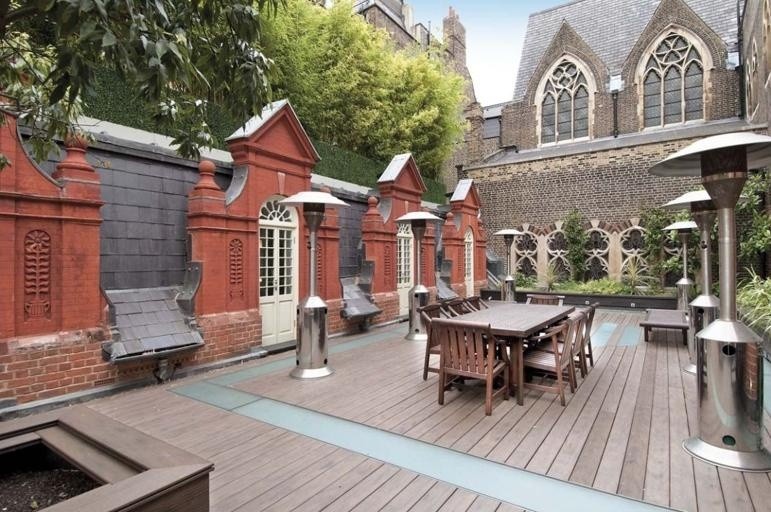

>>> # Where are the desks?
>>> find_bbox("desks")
[444,303,575,406]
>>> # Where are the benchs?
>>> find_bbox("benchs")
[639,309,689,345]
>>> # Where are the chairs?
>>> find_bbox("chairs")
[526,293,565,306]
[429,316,510,417]
[524,301,600,405]
[416,295,489,384]
